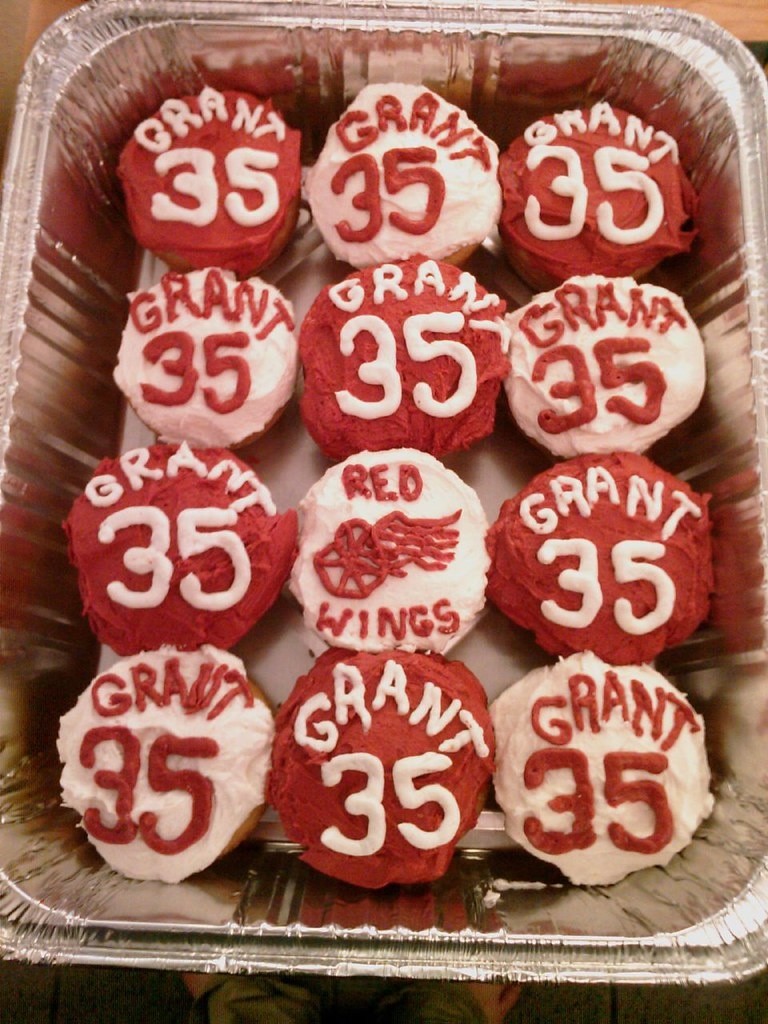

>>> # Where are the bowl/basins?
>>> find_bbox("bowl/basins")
[3,0,768,988]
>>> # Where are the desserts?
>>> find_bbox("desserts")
[54,81,715,886]
